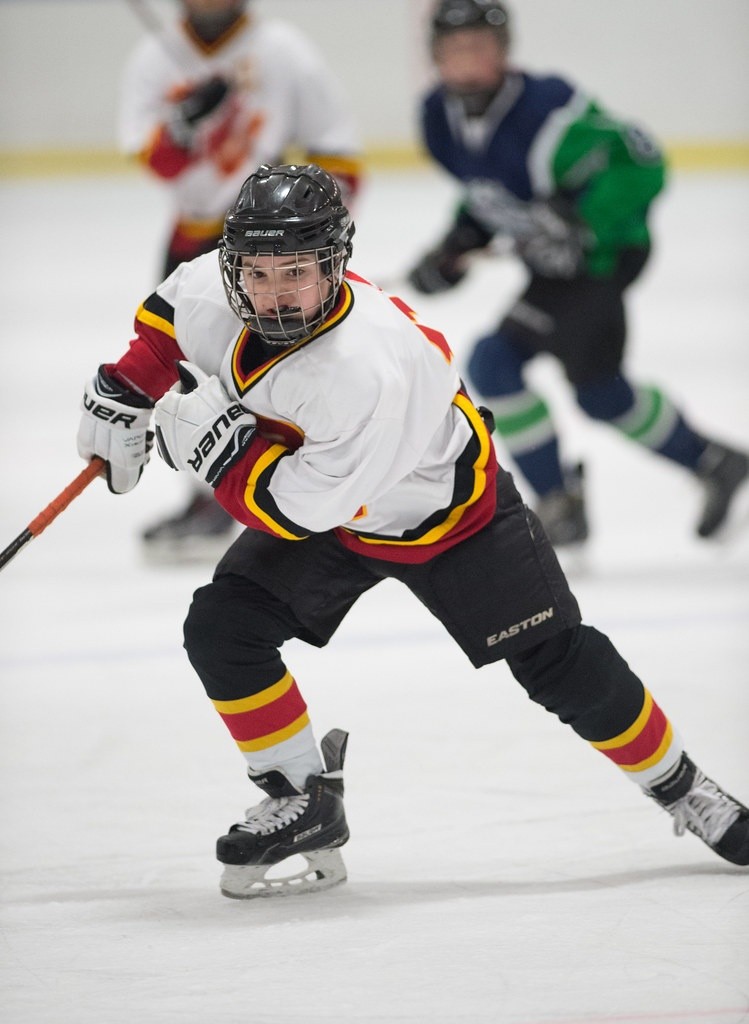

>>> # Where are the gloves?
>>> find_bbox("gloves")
[148,358,258,489]
[531,186,583,246]
[410,225,483,294]
[77,363,154,494]
[167,68,239,133]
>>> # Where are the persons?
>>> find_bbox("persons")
[77,163,749,900]
[408,0,749,547]
[119,1,362,284]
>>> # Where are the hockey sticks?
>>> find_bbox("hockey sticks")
[0,455,107,583]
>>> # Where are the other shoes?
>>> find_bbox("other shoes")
[696,440,748,537]
[535,463,589,544]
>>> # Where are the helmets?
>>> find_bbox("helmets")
[222,162,355,281]
[431,0,508,39]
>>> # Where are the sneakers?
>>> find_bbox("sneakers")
[216,728,349,900]
[639,748,749,866]
